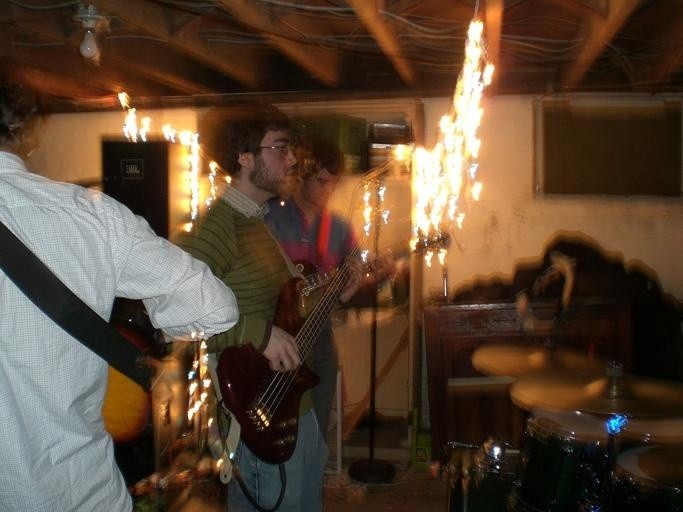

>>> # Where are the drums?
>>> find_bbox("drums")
[523,408,630,510]
[602,446,683,510]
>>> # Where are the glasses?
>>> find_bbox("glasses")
[253,143,294,156]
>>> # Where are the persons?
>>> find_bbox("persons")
[0,64,244,512]
[183,100,364,510]
[264,133,398,436]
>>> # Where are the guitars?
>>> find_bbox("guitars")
[294,223,452,319]
[216,233,371,466]
[103,303,160,441]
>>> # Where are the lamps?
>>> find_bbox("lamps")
[70,1,109,60]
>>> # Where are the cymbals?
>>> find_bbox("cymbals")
[513,376,681,416]
[473,347,631,377]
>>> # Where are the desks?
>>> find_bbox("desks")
[423,289,637,473]
